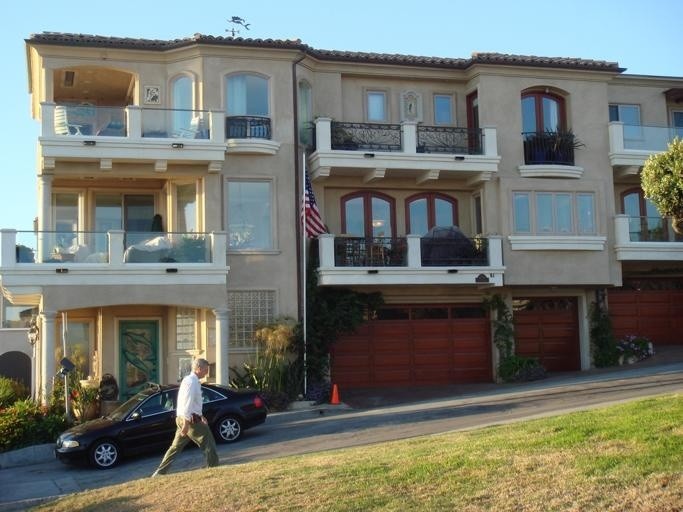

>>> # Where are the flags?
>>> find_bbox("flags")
[300,171,331,239]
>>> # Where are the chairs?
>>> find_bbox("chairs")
[173,116,200,138]
[54,105,83,135]
[164,393,172,411]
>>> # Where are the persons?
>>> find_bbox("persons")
[152,358,218,478]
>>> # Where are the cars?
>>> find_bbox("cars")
[53,382,268,469]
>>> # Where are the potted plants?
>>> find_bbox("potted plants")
[528,127,585,164]
[72,388,99,420]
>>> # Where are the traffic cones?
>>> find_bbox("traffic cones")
[329,384,341,405]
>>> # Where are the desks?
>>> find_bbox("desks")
[50,253,75,263]
[97,128,125,136]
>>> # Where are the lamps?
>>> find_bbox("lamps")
[27,318,39,345]
